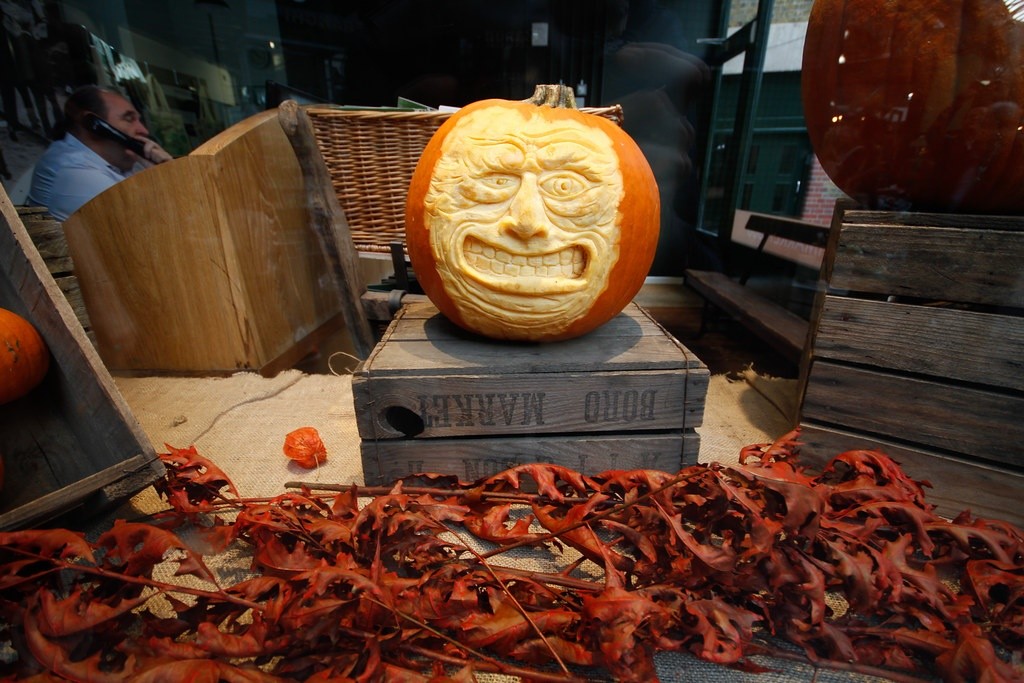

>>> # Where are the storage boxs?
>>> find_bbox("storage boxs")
[0,186,168,530]
[787,201,1024,533]
[352,300,711,486]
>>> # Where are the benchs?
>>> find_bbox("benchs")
[684,215,831,368]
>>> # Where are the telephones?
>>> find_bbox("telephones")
[85,115,152,163]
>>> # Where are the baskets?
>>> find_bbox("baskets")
[300,102,626,253]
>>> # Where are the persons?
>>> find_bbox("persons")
[31,85,173,223]
